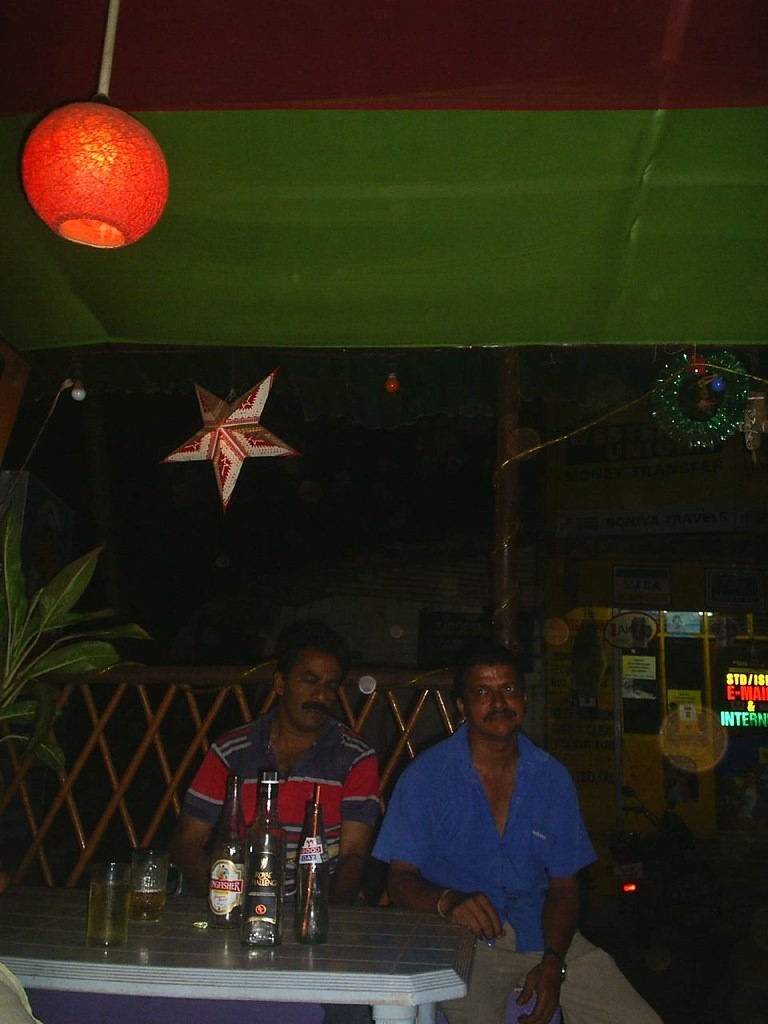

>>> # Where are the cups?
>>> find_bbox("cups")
[86,863,132,951]
[128,850,170,925]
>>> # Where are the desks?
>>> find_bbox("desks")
[1,883,477,1024]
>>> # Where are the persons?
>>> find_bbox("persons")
[365,638,666,1024]
[177,638,376,1024]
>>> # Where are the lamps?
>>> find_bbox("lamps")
[21,0,169,250]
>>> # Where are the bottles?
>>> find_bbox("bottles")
[205,774,249,930]
[241,770,287,947]
[295,801,329,945]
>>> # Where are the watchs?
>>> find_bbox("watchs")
[540,946,567,983]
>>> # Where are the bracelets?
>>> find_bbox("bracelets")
[436,887,455,918]
